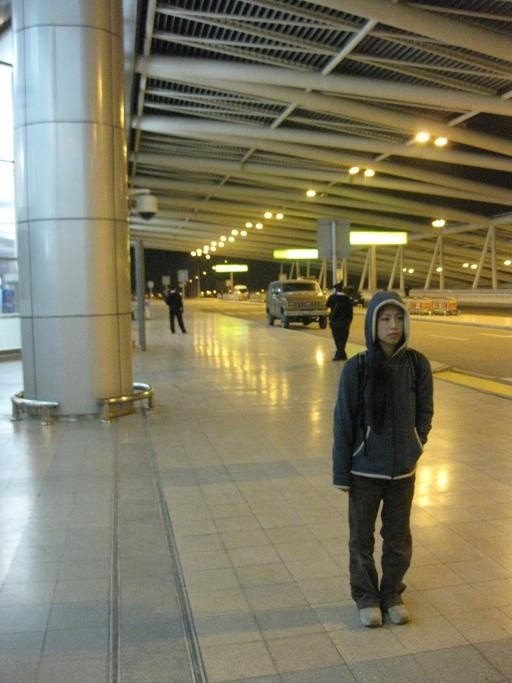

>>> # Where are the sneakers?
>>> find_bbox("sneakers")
[359,608,382,626]
[386,604,410,624]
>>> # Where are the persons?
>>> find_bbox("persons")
[329,290,433,628]
[325,283,353,360]
[165,287,186,333]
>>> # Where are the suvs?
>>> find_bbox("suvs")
[266,278,330,328]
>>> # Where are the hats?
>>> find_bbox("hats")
[330,299,350,322]
